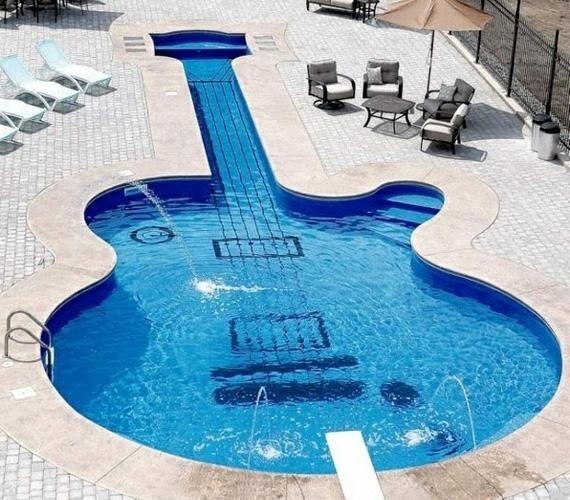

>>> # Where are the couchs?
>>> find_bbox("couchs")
[306,0,367,19]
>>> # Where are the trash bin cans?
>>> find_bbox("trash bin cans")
[532,114,551,152]
[537,122,560,161]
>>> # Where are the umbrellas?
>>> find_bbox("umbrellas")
[374,0,494,97]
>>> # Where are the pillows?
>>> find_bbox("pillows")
[437,85,457,102]
[367,66,383,85]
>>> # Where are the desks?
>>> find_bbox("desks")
[361,94,416,134]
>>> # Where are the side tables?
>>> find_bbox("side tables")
[356,0,380,22]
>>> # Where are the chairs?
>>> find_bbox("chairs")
[307,60,356,111]
[418,103,469,155]
[0,0,89,23]
[0,124,18,144]
[423,78,475,128]
[362,58,403,98]
[0,54,80,112]
[0,99,46,131]
[34,38,113,94]
